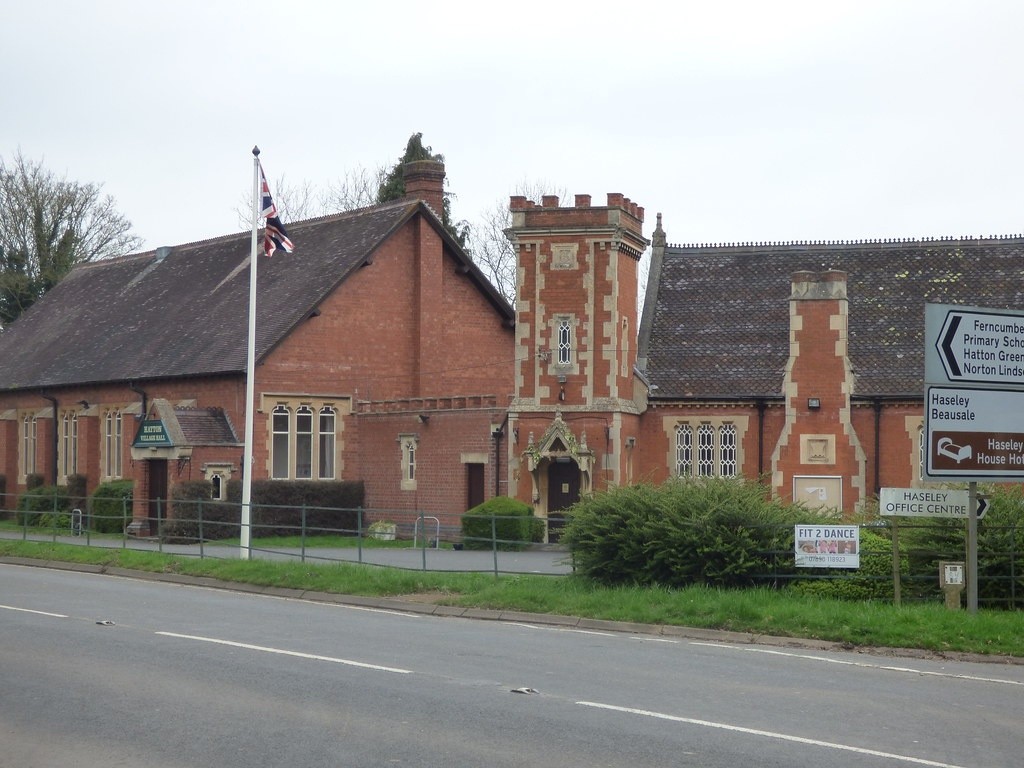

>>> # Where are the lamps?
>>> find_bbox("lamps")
[559,391,565,400]
[808,397,820,408]
[79,401,89,409]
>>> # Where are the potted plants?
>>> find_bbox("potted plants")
[368,520,397,541]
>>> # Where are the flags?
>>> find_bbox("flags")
[258,161,294,258]
